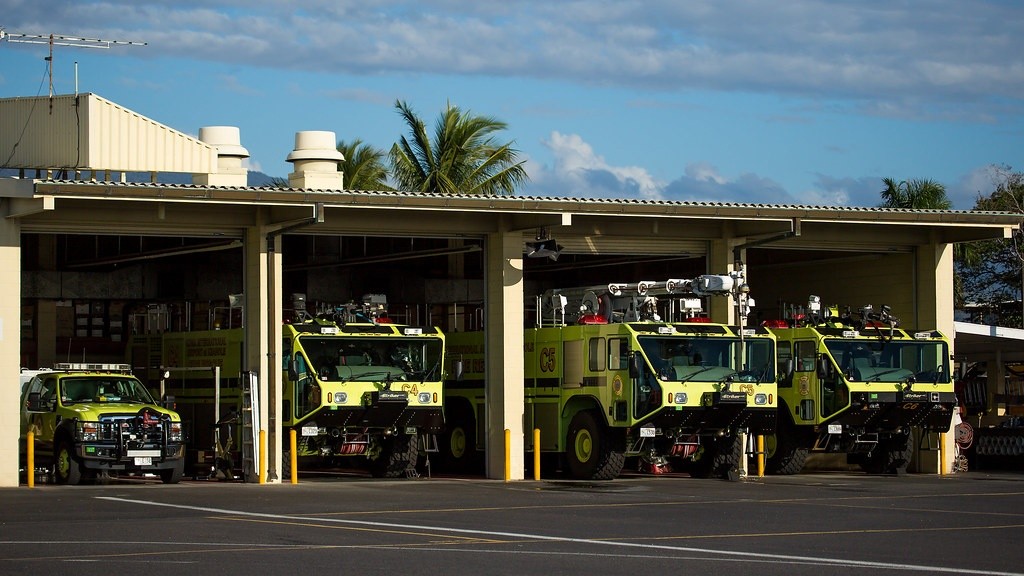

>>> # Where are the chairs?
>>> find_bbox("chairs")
[848,343,874,367]
[672,348,693,367]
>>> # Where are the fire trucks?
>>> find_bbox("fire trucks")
[405,273,777,482]
[123,293,463,480]
[750,293,957,476]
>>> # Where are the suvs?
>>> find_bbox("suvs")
[20,363,184,486]
